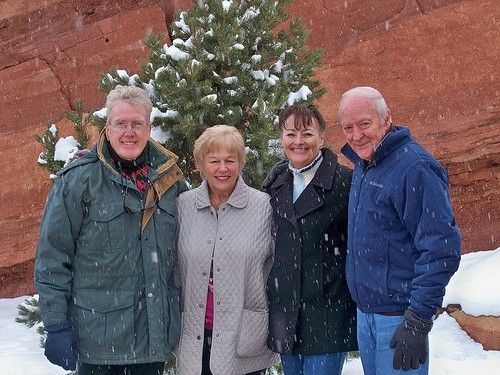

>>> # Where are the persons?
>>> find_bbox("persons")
[172,124,280,375]
[260,102,360,375]
[339,86,463,375]
[34,85,189,375]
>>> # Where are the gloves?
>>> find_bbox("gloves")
[44,329,79,371]
[391,309,432,372]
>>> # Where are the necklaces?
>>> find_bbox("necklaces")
[211,197,218,209]
